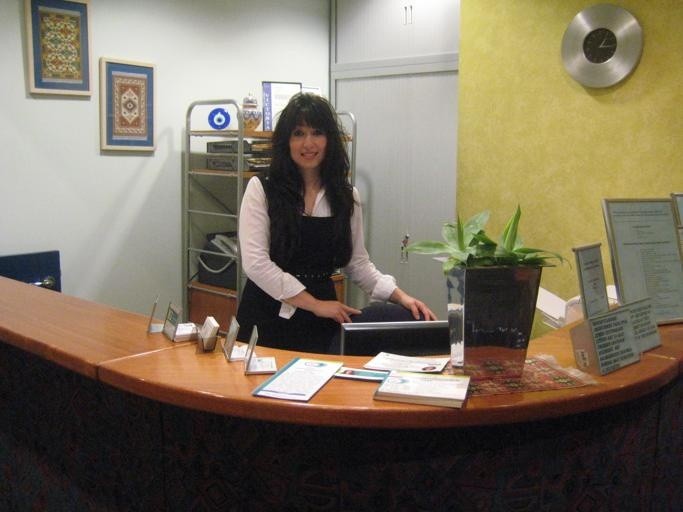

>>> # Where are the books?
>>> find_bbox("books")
[373,368,471,409]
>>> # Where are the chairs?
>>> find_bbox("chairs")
[342,302,425,323]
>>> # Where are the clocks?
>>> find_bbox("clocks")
[560,3,642,88]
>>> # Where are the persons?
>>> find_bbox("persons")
[231,90,439,354]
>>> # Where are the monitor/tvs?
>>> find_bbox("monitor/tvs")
[341,320,452,356]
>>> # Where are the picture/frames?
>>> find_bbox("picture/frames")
[98,57,156,152]
[23,0,92,97]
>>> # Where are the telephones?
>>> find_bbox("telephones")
[211,233,239,263]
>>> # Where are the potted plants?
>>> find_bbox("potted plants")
[401,203,572,382]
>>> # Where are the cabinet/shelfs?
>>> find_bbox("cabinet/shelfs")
[180,98,357,357]
